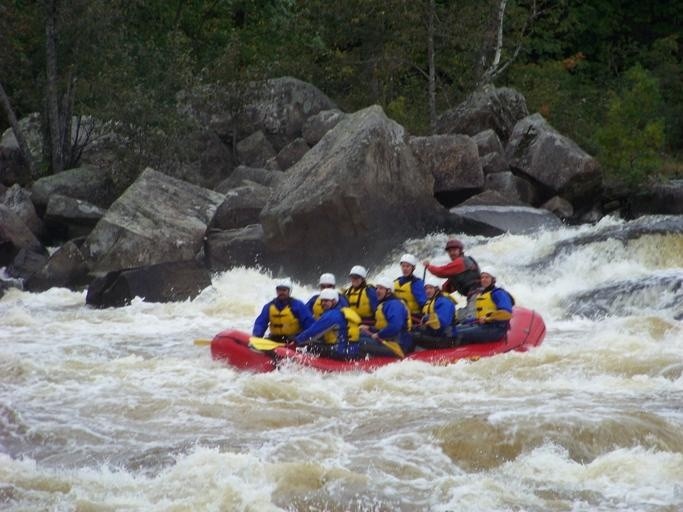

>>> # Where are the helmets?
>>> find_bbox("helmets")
[480,266,496,285]
[276,278,293,297]
[400,254,416,273]
[319,273,335,285]
[349,265,367,279]
[376,273,394,293]
[424,274,442,291]
[320,288,339,306]
[446,240,464,248]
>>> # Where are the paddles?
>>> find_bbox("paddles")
[362,326,403,358]
[250,336,322,353]
[193,337,212,348]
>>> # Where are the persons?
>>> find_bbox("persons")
[281,287,362,361]
[306,272,349,322]
[411,277,456,349]
[342,264,377,327]
[252,280,314,342]
[454,266,516,347]
[359,278,411,358]
[393,253,426,325]
[423,239,480,304]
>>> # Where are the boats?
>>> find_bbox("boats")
[209,304,547,375]
